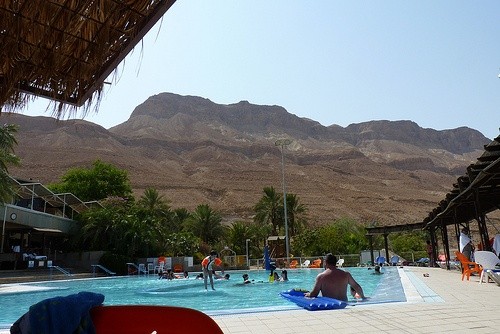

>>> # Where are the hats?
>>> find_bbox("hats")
[225,274,230,278]
[242,274,248,278]
[209,250,217,255]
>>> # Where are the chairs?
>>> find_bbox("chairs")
[473,250,500,284]
[453,251,482,282]
[309,259,322,268]
[174,264,183,271]
[301,259,311,269]
[336,259,344,268]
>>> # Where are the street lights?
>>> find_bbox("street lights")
[273,137,292,270]
[245,238,251,267]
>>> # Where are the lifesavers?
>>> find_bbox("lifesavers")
[173,265,182,273]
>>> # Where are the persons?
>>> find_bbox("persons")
[201,249,222,291]
[243,273,251,284]
[158,268,230,280]
[350,286,360,299]
[273,269,289,283]
[459,227,478,276]
[425,240,435,267]
[371,266,383,274]
[304,253,365,301]
